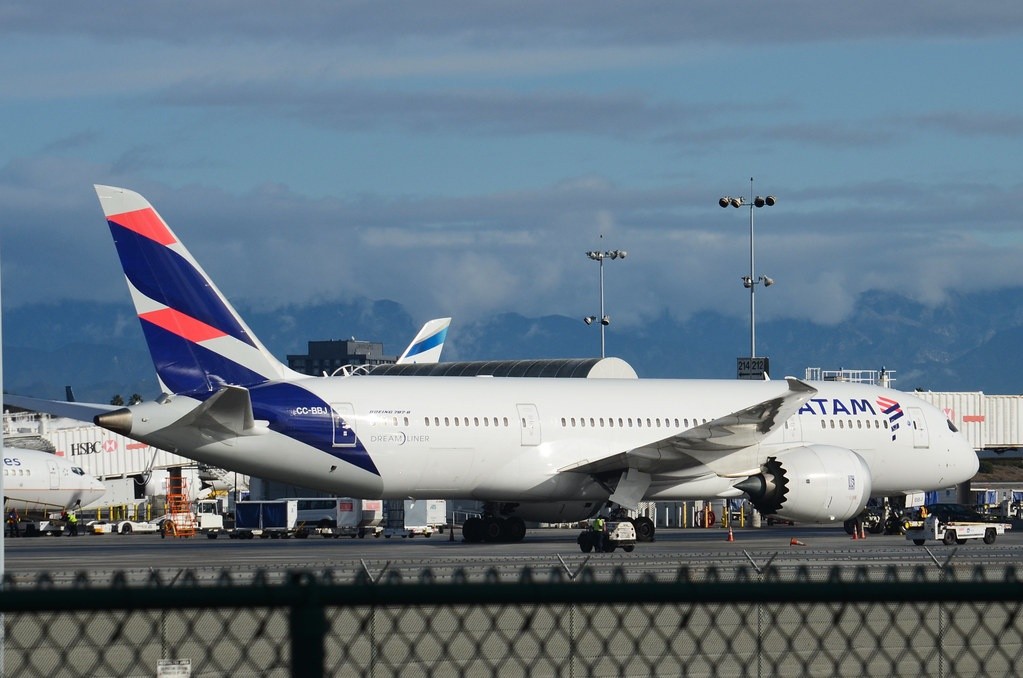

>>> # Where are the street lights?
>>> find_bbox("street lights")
[583,249,626,360]
[717,178,775,358]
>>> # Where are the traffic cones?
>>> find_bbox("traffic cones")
[726,526,736,542]
[790,537,806,546]
[852,524,866,540]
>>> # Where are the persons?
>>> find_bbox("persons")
[60,512,78,536]
[592,515,604,554]
[7,509,20,537]
[855,512,865,534]
[919,505,928,521]
[160,520,167,539]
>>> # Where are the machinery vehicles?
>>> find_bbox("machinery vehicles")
[576,519,638,552]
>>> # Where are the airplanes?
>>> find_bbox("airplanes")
[3,445,108,520]
[3,183,981,552]
